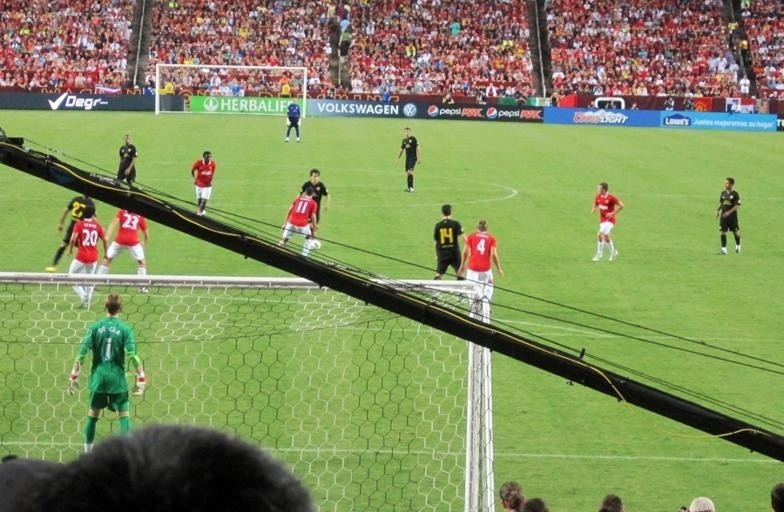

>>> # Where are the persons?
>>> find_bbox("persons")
[716,178,744,256]
[0,0,782,114]
[191,151,214,216]
[590,182,623,261]
[458,219,503,317]
[397,128,420,191]
[499,480,782,511]
[284,98,301,143]
[116,135,137,184]
[44,194,95,272]
[299,169,330,237]
[67,206,110,307]
[70,293,146,453]
[276,187,316,257]
[96,209,149,294]
[432,204,466,280]
[0,423,313,511]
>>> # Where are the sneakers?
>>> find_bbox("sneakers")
[718,250,727,255]
[609,250,619,260]
[592,252,603,261]
[735,245,740,254]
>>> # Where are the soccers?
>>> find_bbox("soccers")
[309,240,319,251]
